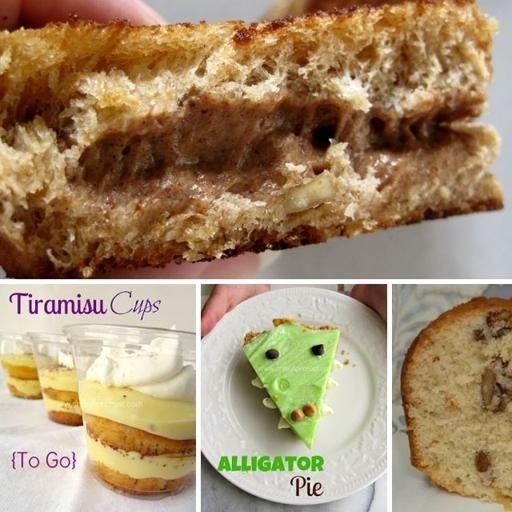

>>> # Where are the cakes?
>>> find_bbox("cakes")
[0,317,199,501]
[239,317,342,451]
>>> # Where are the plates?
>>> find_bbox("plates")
[199,288,390,506]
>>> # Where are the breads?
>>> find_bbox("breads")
[400,296,511,510]
[1,1,506,275]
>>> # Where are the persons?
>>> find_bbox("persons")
[0,1,277,279]
[201,284,387,338]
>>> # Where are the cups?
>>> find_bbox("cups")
[2,319,197,503]
